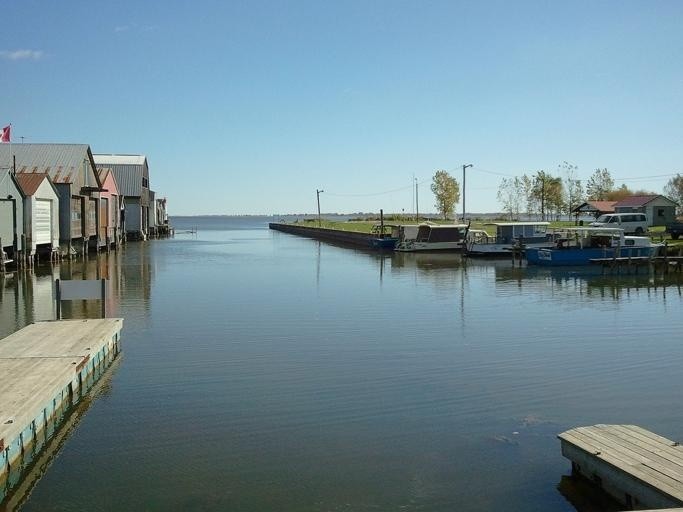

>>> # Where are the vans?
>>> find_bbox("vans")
[590,212,647,235]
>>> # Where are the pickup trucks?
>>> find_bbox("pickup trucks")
[666,221,683,240]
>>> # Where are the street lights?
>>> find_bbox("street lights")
[463,164,472,223]
[317,189,324,227]
[415,178,418,223]
[532,175,544,220]
